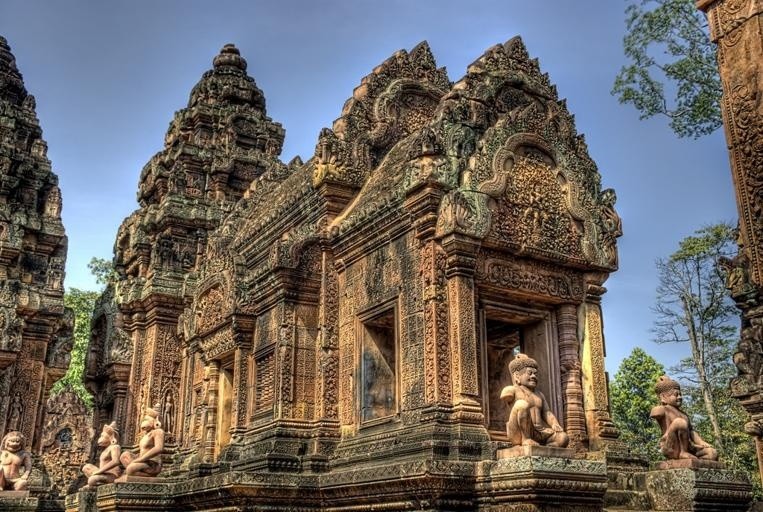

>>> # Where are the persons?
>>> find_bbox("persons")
[79,402,165,490]
[162,393,174,433]
[7,392,23,433]
[1,433,34,492]
[647,374,720,460]
[499,352,570,448]
